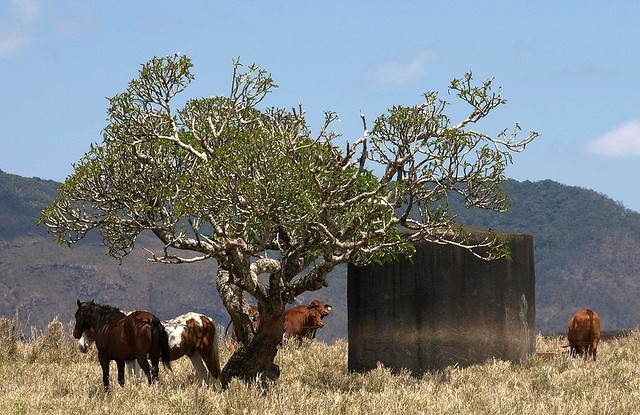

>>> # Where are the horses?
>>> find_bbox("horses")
[73,299,173,390]
[78,310,221,383]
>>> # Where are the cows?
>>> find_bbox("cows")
[283,310,324,347]
[561,309,600,360]
[223,305,262,348]
[307,300,333,339]
